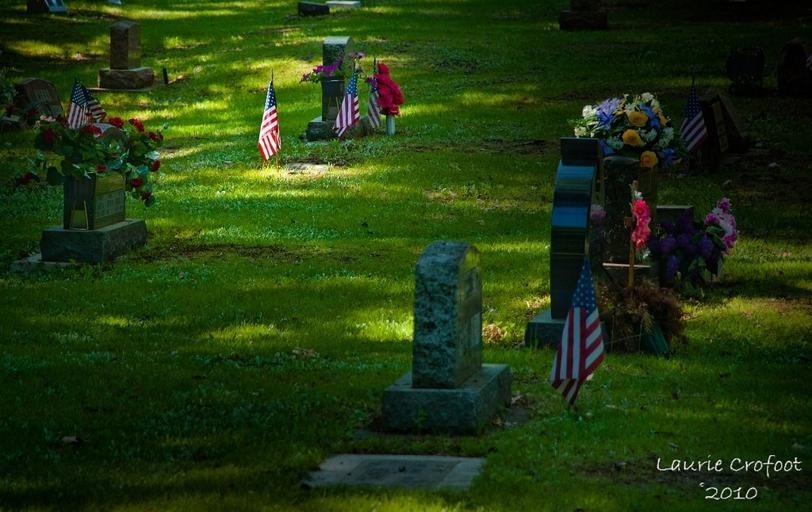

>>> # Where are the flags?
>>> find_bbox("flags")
[678,83,710,155]
[549,254,605,407]
[331,73,359,140]
[366,73,383,130]
[257,80,281,161]
[81,83,107,123]
[66,81,92,130]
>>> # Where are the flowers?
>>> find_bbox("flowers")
[33,104,163,205]
[565,91,739,299]
[300,51,376,88]
